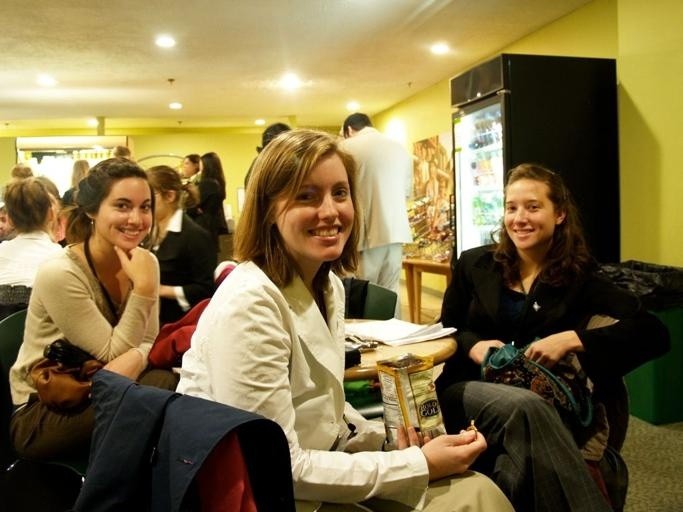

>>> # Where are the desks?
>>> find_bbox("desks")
[344,318,457,382]
[402,258,452,324]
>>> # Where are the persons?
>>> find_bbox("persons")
[7,156,177,464]
[244,113,413,320]
[173,128,517,512]
[433,161,671,512]
[1,145,229,325]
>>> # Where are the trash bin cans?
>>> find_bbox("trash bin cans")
[598,259,683,426]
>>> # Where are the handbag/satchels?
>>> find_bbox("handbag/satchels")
[29,337,106,410]
[479,337,597,439]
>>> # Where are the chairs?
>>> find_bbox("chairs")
[341,278,398,320]
[605,446,629,512]
[0,309,89,477]
[103,375,258,512]
